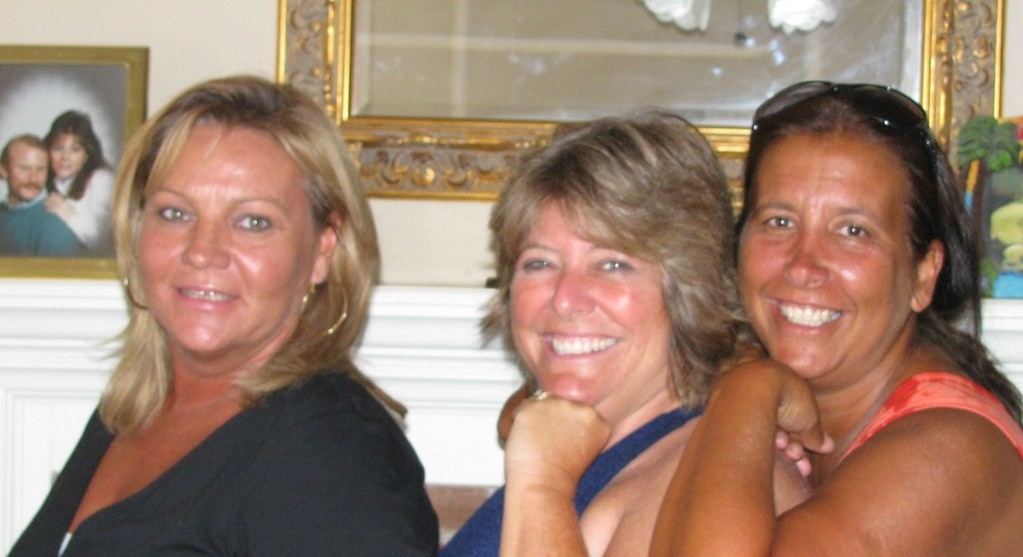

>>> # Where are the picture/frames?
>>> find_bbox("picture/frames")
[0,48,148,279]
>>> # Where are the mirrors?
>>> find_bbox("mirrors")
[276,0,1004,214]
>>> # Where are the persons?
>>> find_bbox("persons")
[651,79,1023,557]
[427,109,814,557]
[9,78,441,557]
[0,110,120,257]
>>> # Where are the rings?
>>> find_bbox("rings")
[532,385,551,399]
